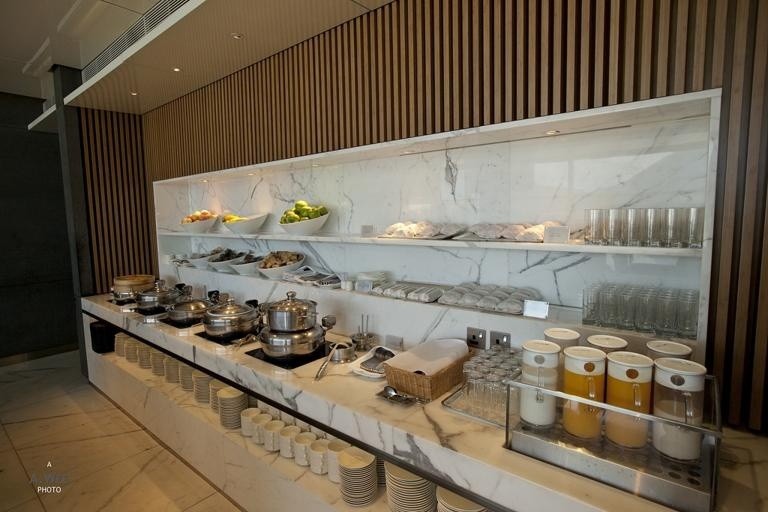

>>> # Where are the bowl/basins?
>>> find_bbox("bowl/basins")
[351,332,376,352]
[329,341,356,363]
[180,210,331,236]
[189,248,305,280]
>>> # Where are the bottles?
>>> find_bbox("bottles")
[341,280,353,291]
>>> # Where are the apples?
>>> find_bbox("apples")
[279,200,327,224]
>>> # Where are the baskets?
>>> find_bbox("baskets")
[382,346,474,403]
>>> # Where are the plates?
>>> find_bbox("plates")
[349,346,398,379]
[337,446,491,512]
[115,332,249,429]
[298,275,341,289]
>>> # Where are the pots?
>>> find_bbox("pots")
[111,288,142,301]
[136,278,262,338]
[257,291,336,359]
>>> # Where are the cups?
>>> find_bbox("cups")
[581,208,702,337]
[464,344,523,418]
[521,329,707,461]
[165,253,187,266]
[240,400,352,483]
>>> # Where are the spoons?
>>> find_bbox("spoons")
[384,386,424,401]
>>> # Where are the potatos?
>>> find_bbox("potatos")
[182,209,213,224]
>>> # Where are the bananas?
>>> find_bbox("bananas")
[222,214,248,223]
